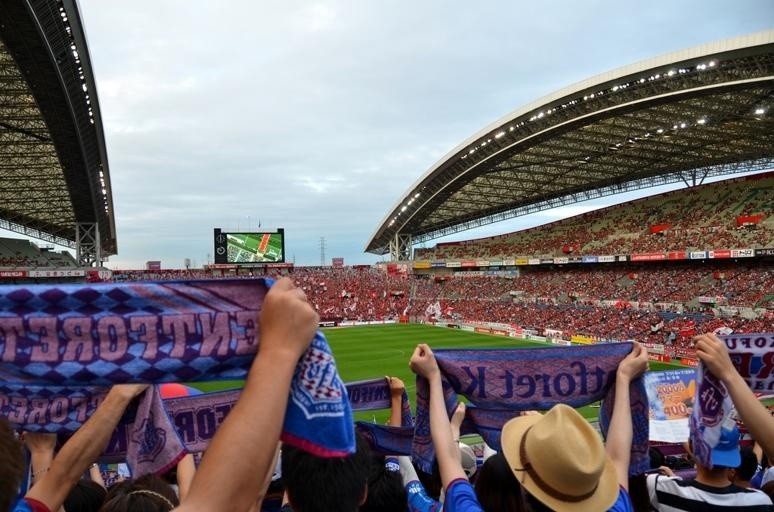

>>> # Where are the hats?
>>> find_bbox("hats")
[457,441,478,478]
[501,403,623,512]
[687,413,743,470]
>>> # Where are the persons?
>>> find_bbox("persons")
[0,175,774,511]
